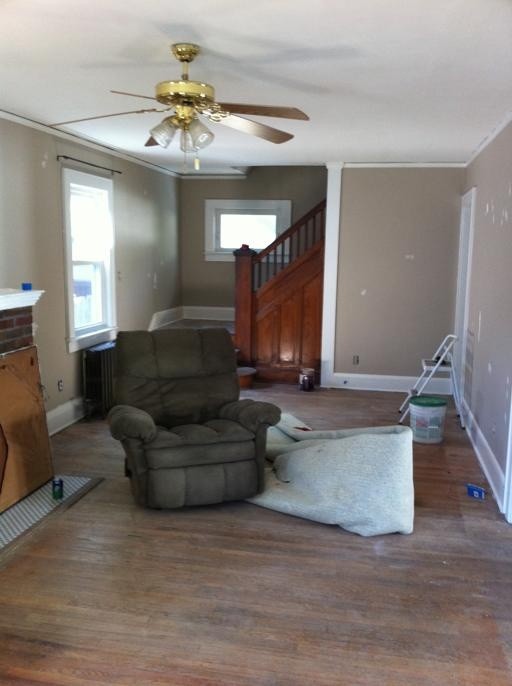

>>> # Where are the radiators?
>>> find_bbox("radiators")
[85,342,117,421]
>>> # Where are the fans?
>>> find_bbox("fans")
[47,42,309,144]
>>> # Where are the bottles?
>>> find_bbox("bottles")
[298,368,315,392]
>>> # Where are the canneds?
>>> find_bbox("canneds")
[52,478,63,499]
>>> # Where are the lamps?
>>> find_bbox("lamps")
[144,110,214,174]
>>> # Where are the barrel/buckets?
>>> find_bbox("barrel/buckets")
[408,396,446,444]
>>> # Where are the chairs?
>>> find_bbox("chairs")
[107,327,282,511]
